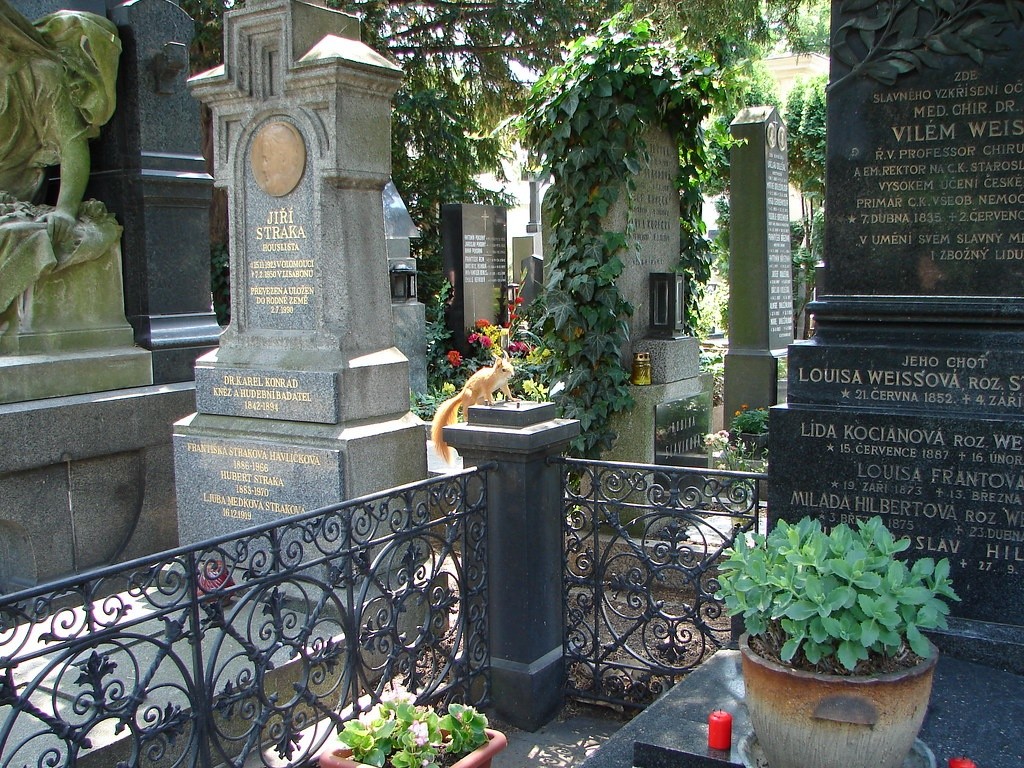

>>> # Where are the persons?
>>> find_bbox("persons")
[0,0,119,333]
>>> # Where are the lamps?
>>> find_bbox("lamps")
[390,261,418,302]
[508,282,522,313]
[647,271,691,342]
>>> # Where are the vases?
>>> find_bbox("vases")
[320,728,508,768]
[728,429,769,461]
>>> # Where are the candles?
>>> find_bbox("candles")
[709,708,732,751]
[947,754,977,768]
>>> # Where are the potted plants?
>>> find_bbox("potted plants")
[718,513,964,768]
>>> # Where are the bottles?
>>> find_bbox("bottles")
[633,352,652,385]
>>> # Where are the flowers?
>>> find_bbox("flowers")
[335,679,490,768]
[731,404,769,434]
